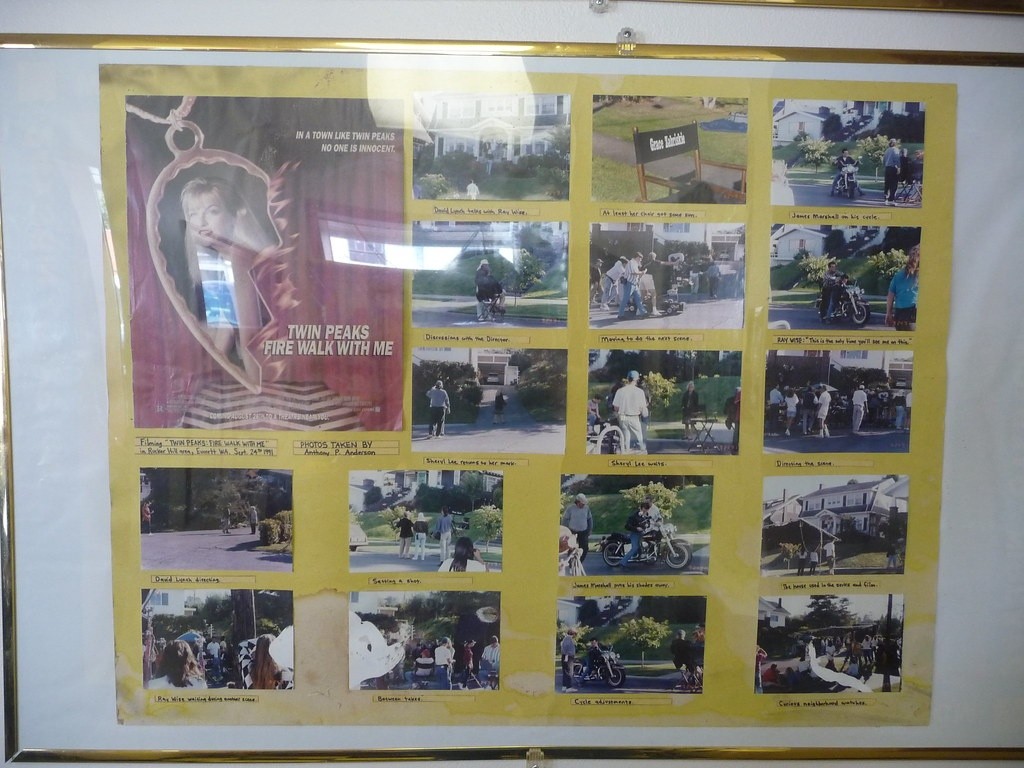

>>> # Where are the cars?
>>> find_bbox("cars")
[349,525,369,552]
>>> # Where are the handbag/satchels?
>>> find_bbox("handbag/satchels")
[432,531,441,540]
[620,276,627,284]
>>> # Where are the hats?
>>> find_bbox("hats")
[469,640,477,644]
[628,370,639,380]
[481,259,489,264]
[490,635,498,642]
[620,256,628,264]
[436,381,443,387]
[596,259,603,263]
[891,138,896,142]
[422,650,431,657]
[575,494,588,504]
[442,637,450,643]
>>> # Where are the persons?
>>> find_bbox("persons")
[493,387,508,424]
[221,502,257,533]
[144,634,291,690]
[141,501,154,532]
[173,176,364,432]
[820,244,920,331]
[830,149,865,196]
[475,259,505,322]
[796,537,836,576]
[369,629,501,690]
[756,634,902,694]
[883,139,924,206]
[425,380,450,439]
[770,380,912,440]
[562,492,663,570]
[886,537,898,569]
[590,251,722,319]
[466,179,480,199]
[587,370,741,451]
[395,505,491,572]
[559,622,705,691]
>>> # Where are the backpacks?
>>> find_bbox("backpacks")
[854,642,863,655]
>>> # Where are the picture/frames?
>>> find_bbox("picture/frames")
[0,31,1024,767]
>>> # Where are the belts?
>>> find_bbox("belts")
[605,274,615,284]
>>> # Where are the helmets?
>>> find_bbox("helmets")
[568,629,578,634]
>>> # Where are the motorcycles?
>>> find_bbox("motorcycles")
[831,156,863,198]
[597,517,692,571]
[574,643,626,689]
[816,280,870,327]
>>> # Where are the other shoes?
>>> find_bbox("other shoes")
[886,200,897,205]
[825,318,831,324]
[651,312,662,316]
[562,687,566,691]
[567,688,577,692]
